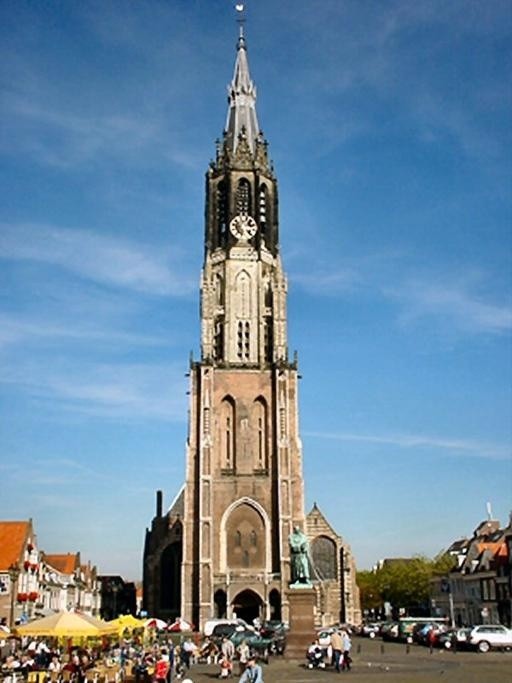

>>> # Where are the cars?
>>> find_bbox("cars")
[203,619,289,664]
[362,617,511,653]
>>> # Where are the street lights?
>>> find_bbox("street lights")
[8,562,20,628]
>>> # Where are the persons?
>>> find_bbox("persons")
[0,605,262,683]
[305,627,353,673]
[286,526,311,584]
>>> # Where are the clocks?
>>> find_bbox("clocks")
[228,214,259,244]
